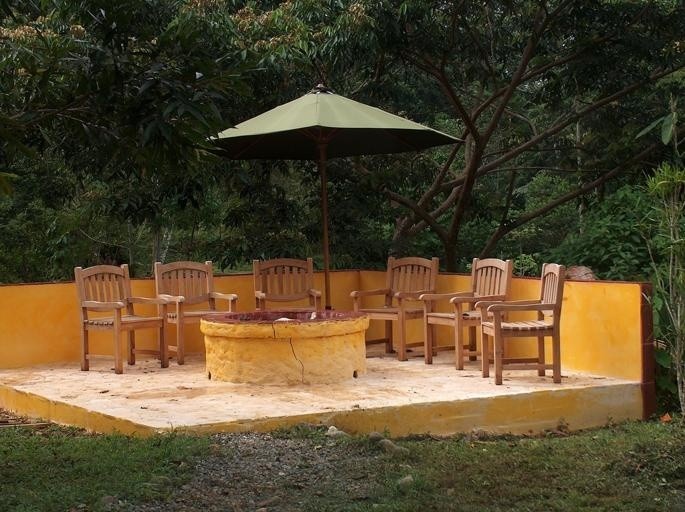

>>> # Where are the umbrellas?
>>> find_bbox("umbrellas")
[198,84,467,309]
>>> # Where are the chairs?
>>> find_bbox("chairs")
[153,261,237,365]
[475,262,565,384]
[419,257,512,370]
[253,258,321,311]
[75,264,167,374]
[349,254,438,363]
